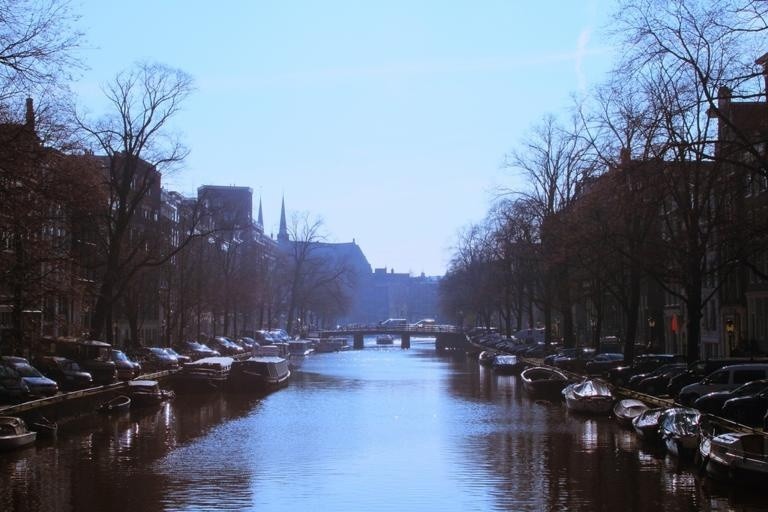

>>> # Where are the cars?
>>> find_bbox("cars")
[334,325,343,330]
[469,323,767,430]
[0,310,291,399]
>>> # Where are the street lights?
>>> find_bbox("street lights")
[646,314,657,349]
[591,317,596,348]
[724,318,737,359]
[554,315,562,347]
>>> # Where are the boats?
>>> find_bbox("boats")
[377,335,393,344]
[694,423,768,491]
[656,406,702,457]
[310,337,350,351]
[288,338,317,358]
[0,392,129,454]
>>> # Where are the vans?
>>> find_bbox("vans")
[377,319,406,328]
[409,319,435,331]
